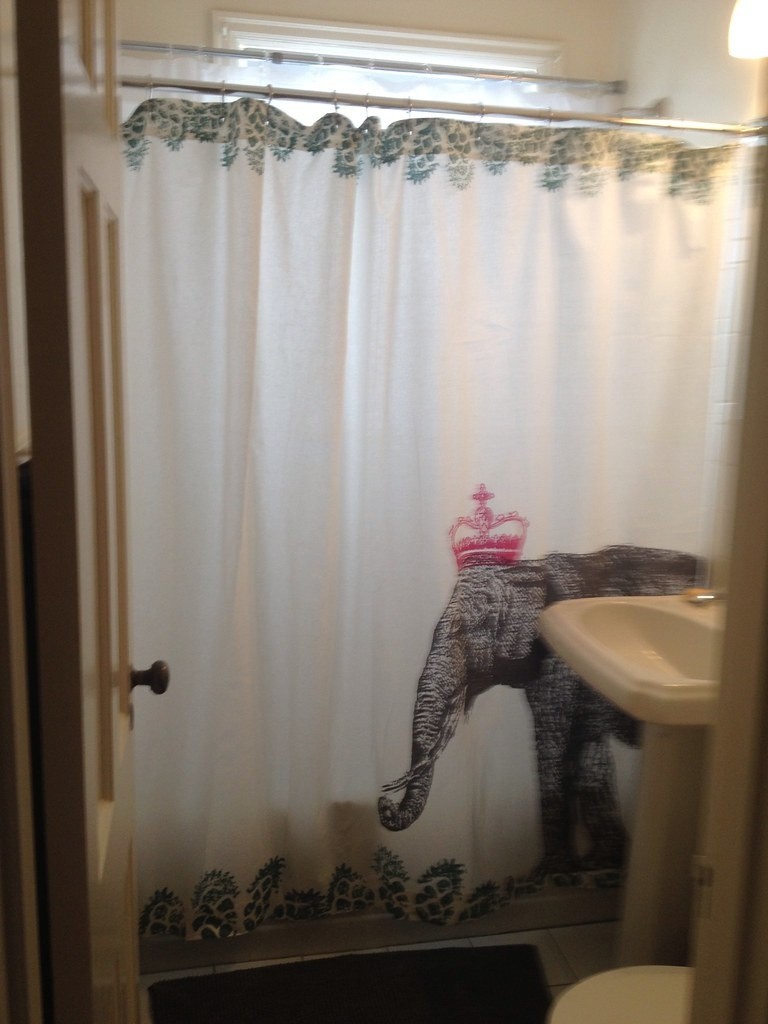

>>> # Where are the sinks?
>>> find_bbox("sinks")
[539,594,721,727]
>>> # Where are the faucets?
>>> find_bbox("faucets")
[684,589,728,607]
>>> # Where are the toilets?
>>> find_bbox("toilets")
[544,965,692,1023]
